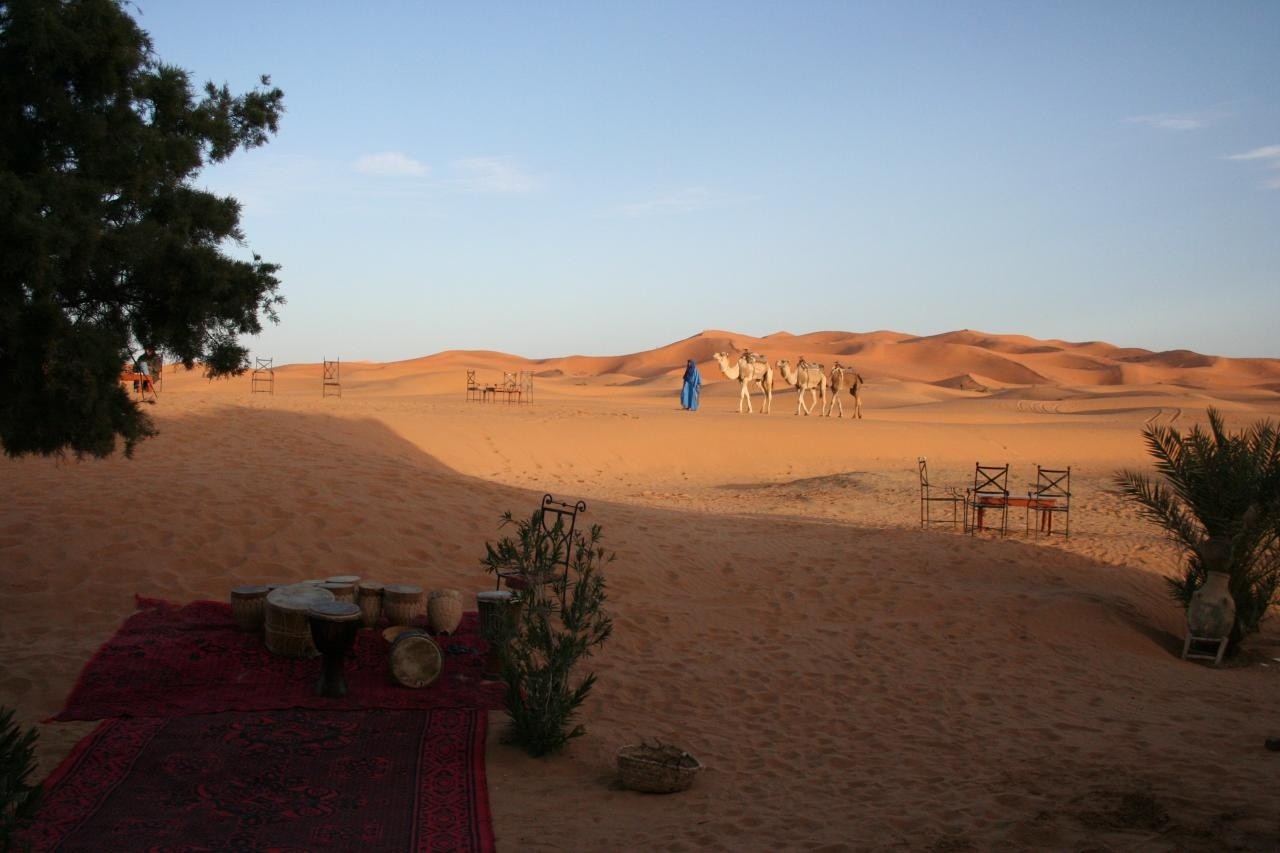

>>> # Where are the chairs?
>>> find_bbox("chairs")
[251,358,275,394]
[157,353,163,391]
[322,355,342,400]
[965,462,1009,540]
[465,370,535,405]
[1024,464,1073,541]
[496,494,587,610]
[916,455,967,534]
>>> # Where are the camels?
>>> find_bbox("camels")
[775,358,828,416]
[826,361,864,418]
[712,351,775,415]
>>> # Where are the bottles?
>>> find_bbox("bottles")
[1188,571,1235,639]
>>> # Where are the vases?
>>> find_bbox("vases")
[1186,571,1236,636]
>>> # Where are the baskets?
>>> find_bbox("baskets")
[615,744,701,792]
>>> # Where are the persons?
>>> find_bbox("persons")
[681,359,702,410]
[133,347,162,388]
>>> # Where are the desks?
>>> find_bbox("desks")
[477,385,526,404]
[976,491,1058,535]
[118,372,154,393]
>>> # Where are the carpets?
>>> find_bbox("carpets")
[0,708,497,853]
[40,591,530,719]
